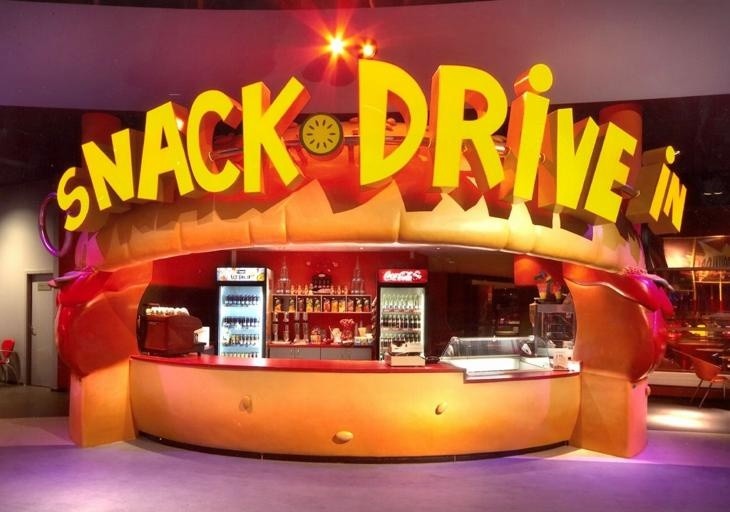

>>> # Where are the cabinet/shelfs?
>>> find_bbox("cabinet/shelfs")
[382,308,421,354]
[271,293,372,346]
[437,333,553,358]
[224,303,259,358]
[269,342,373,361]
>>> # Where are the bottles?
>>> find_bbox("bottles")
[289,275,349,296]
[273,297,369,313]
[222,293,260,357]
[380,292,420,358]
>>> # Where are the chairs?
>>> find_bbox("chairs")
[0,337,19,387]
[653,313,730,411]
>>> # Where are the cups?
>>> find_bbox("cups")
[358,327,366,337]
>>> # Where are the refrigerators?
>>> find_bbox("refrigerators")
[376,266,429,365]
[215,265,273,361]
